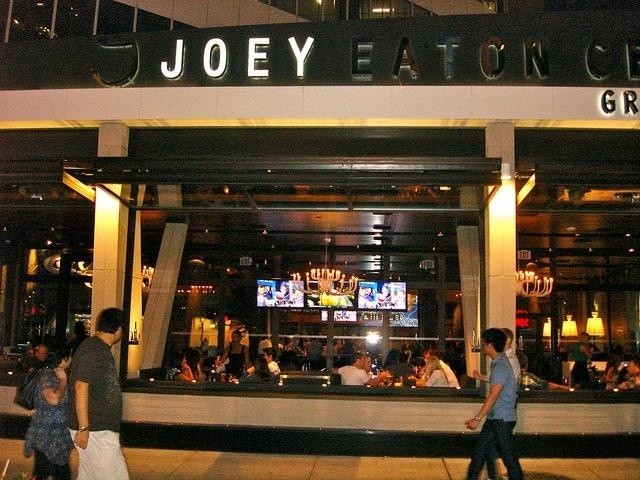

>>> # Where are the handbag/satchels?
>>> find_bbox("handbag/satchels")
[13,363,58,411]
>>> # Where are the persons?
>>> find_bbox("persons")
[20,346,47,372]
[258,330,371,365]
[333,344,459,387]
[67,307,130,480]
[518,331,639,390]
[402,294,418,319]
[464,328,524,480]
[471,328,522,480]
[68,321,90,350]
[24,342,79,479]
[167,332,284,386]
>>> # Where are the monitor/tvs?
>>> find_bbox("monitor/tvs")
[358,310,384,327]
[388,288,420,328]
[306,280,359,310]
[320,310,357,324]
[357,281,408,311]
[255,278,305,310]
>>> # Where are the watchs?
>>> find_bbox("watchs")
[79,426,90,432]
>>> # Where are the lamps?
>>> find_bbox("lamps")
[516,268,555,299]
[584,311,606,337]
[542,317,552,339]
[560,314,579,337]
[290,234,360,296]
